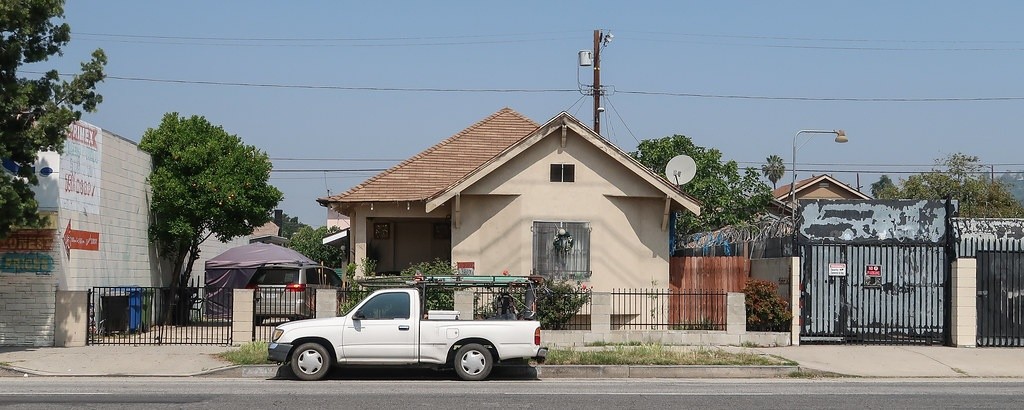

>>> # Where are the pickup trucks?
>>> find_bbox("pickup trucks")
[267,272,550,383]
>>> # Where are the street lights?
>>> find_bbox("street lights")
[792,129,850,245]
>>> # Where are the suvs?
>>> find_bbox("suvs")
[256,264,350,325]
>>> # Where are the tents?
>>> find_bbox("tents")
[203,242,344,320]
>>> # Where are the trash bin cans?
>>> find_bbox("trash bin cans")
[101,291,130,336]
[111,285,143,334]
[142,289,155,332]
